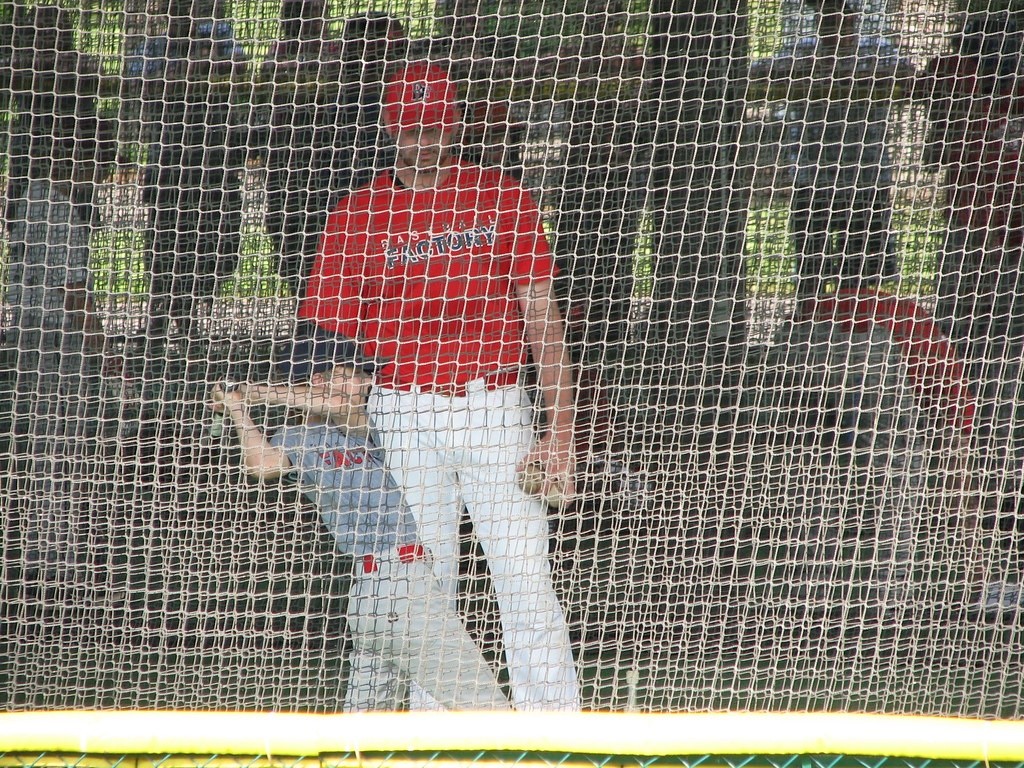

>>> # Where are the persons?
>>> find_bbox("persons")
[207,329,510,710]
[0,0,1024,605]
[301,64,580,711]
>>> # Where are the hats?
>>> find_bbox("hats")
[278,330,392,386]
[33,109,134,167]
[463,100,528,136]
[385,58,458,134]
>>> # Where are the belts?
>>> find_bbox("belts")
[364,541,422,574]
[376,374,523,398]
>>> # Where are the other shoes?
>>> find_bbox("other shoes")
[34,574,128,610]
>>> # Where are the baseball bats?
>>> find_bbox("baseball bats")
[208,376,238,455]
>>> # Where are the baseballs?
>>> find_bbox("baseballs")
[518,465,545,495]
[544,479,568,508]
